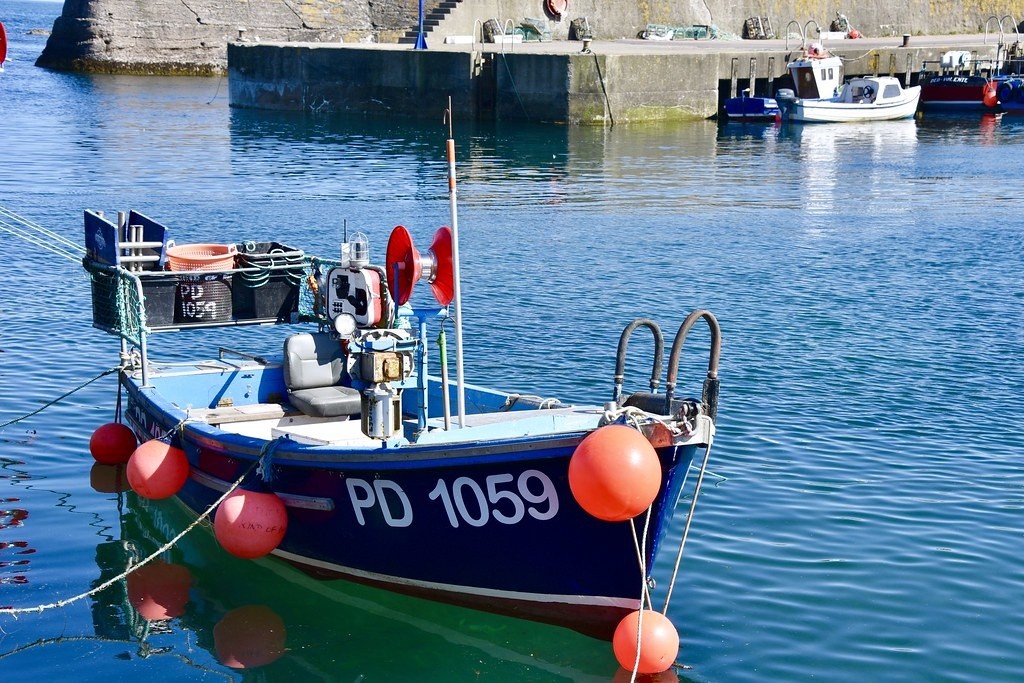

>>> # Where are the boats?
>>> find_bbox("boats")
[722,13,1024,124]
[82,93,723,676]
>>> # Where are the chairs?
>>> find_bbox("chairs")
[284,333,362,417]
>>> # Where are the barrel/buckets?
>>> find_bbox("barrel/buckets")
[235,241,305,320]
[139,277,179,327]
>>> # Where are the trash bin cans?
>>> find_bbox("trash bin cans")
[165,242,237,326]
